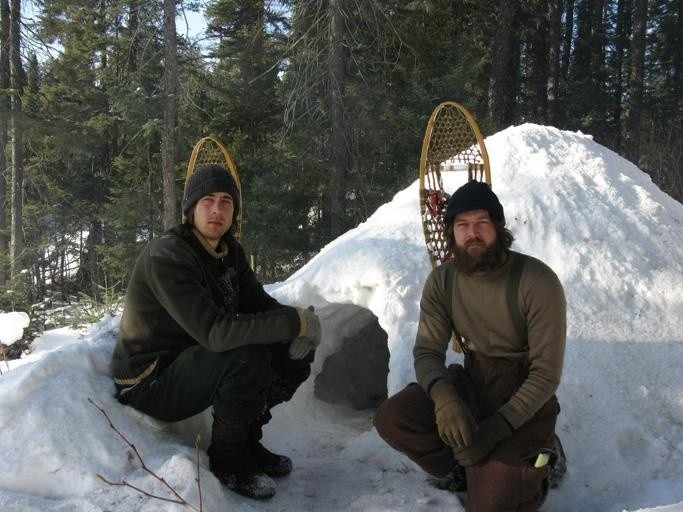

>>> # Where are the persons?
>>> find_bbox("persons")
[372,179,567,511]
[110,163,323,500]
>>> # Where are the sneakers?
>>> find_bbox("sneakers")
[245,443,292,478]
[208,458,279,500]
[428,466,467,492]
[548,435,568,491]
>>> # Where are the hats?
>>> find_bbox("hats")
[444,180,506,228]
[182,164,241,218]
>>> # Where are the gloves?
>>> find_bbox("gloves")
[452,411,514,470]
[288,305,322,361]
[429,378,479,449]
